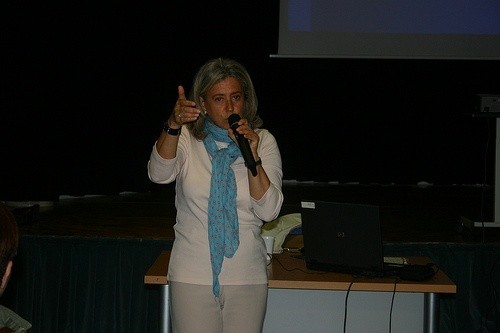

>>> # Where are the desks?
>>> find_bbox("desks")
[144,234,456,333]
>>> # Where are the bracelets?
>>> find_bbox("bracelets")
[163,122,181,136]
[245,157,261,167]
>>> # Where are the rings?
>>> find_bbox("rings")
[178,113,182,118]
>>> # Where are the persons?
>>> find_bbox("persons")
[0,202,32,333]
[147,58,285,333]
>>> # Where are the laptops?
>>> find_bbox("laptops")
[301,200,406,278]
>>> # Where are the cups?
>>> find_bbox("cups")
[261,236,275,257]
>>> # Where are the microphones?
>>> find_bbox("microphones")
[228,114,258,177]
